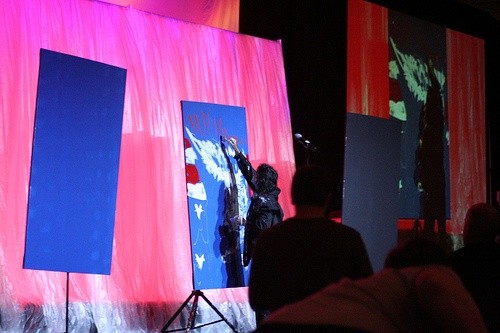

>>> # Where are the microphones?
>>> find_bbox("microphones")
[294,133,318,153]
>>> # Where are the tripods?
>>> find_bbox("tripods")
[409,218,423,235]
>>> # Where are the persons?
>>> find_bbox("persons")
[228,137,284,326]
[452,202,500,333]
[255,233,487,333]
[248,164,373,318]
